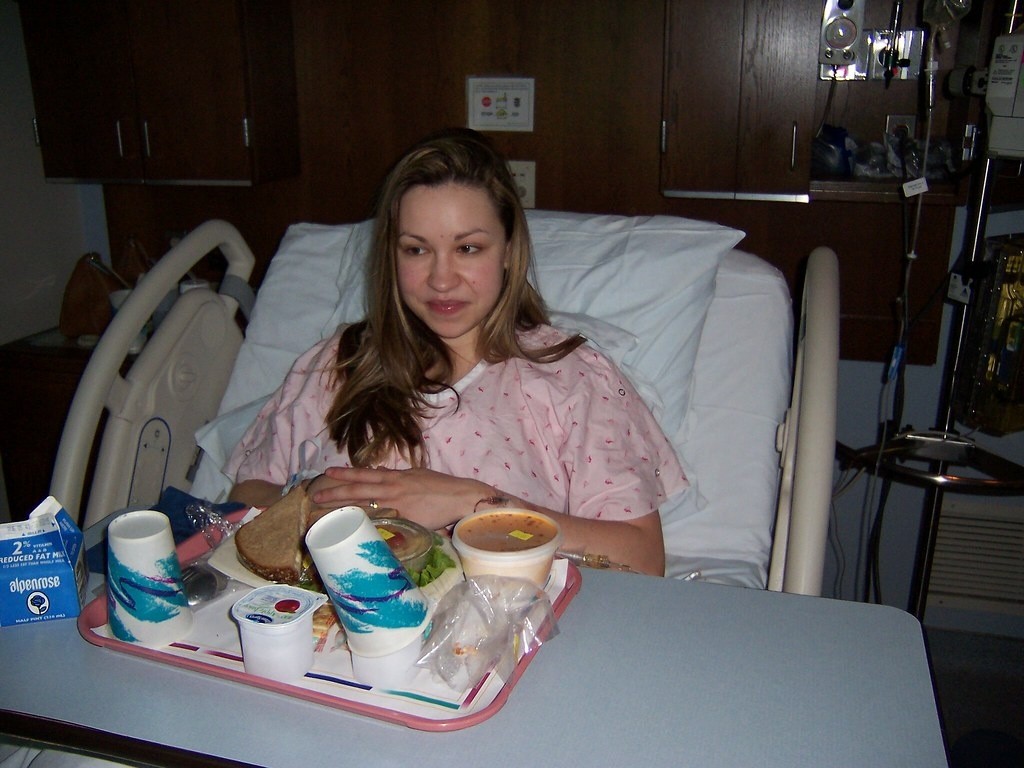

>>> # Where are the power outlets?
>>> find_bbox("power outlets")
[505,161,536,210]
[887,114,917,139]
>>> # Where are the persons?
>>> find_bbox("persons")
[226,138,690,576]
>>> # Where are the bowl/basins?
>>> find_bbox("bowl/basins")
[452,508,563,599]
[369,517,435,573]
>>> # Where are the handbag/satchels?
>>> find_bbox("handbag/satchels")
[61,234,149,338]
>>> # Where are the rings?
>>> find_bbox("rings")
[370,497,377,509]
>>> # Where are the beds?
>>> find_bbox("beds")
[0,222,841,768]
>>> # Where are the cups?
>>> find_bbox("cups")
[110,290,149,355]
[106,509,193,647]
[304,506,436,658]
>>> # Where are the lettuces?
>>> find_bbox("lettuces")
[393,531,457,587]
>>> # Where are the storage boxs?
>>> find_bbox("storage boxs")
[0,495,90,627]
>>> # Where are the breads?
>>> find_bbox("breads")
[235,488,397,585]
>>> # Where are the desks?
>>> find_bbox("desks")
[0,506,950,768]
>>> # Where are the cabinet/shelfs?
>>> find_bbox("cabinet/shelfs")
[1,326,135,527]
[19,0,1023,363]
